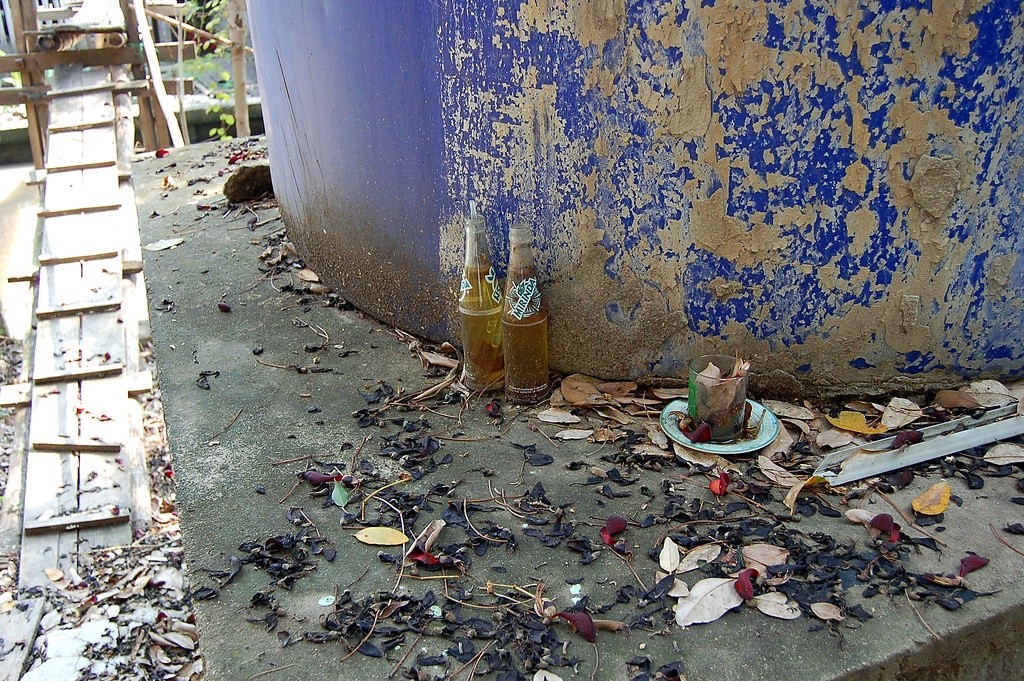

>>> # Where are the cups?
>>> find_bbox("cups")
[689,354,748,439]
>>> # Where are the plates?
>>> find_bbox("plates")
[659,397,780,454]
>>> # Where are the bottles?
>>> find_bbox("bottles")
[458,214,505,392]
[501,225,548,404]
